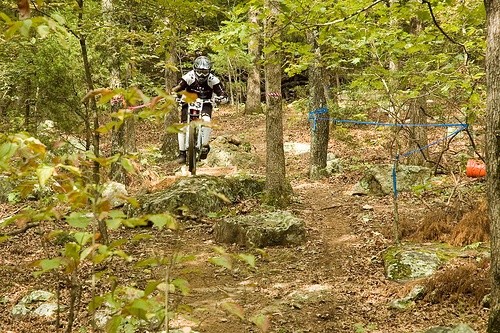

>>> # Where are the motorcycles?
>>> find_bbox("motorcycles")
[170,94,221,175]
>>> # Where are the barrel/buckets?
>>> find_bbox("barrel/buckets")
[466,155,487,176]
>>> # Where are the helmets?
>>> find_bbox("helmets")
[193,55,211,70]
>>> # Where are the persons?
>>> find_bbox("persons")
[170,56,229,165]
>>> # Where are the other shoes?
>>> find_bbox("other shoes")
[178,155,186,164]
[200,143,210,159]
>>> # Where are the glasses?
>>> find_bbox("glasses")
[196,69,210,77]
